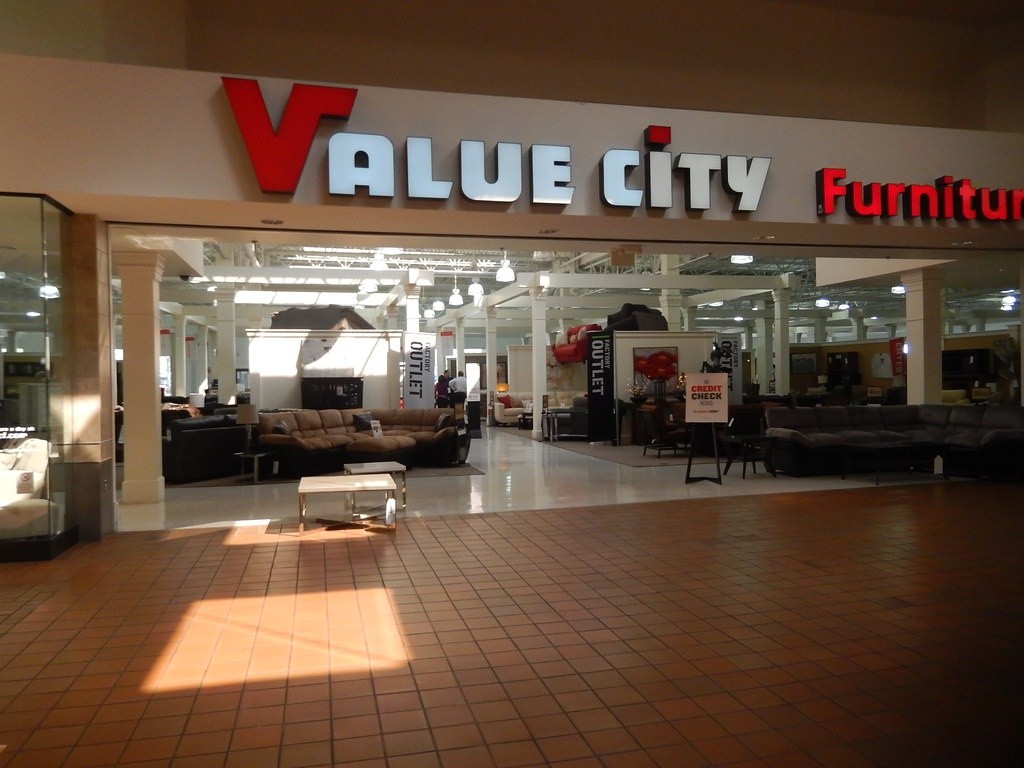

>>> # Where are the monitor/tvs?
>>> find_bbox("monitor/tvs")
[300,376,363,410]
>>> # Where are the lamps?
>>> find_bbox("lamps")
[449,274,464,306]
[468,269,484,296]
[370,253,388,269]
[816,300,830,308]
[709,301,723,307]
[839,304,850,310]
[495,251,516,282]
[237,403,261,453]
[730,255,754,265]
[533,251,553,262]
[891,285,905,294]
[39,285,60,299]
[424,309,435,318]
[432,300,446,311]
[1000,293,1016,310]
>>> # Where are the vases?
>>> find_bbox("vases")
[630,397,648,405]
[674,390,686,403]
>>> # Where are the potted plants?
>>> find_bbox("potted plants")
[611,398,628,446]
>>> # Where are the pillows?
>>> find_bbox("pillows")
[434,413,451,433]
[497,395,512,409]
[275,419,302,438]
[352,411,374,431]
[521,400,533,407]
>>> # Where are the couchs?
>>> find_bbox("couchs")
[162,414,259,484]
[0,437,59,538]
[763,404,1024,479]
[257,407,471,476]
[494,391,589,436]
[692,404,767,458]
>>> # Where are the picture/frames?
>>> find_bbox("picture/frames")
[633,346,678,395]
[480,362,507,390]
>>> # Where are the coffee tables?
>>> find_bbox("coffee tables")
[346,435,416,472]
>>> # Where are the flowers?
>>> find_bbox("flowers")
[676,372,687,390]
[624,371,651,397]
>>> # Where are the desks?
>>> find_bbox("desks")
[540,409,588,442]
[719,434,780,479]
[298,473,397,537]
[343,461,407,514]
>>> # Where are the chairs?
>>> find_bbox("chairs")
[637,405,688,459]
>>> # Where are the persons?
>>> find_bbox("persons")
[436,375,451,408]
[444,369,454,381]
[448,371,467,407]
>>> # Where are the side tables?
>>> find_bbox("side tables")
[234,452,266,483]
